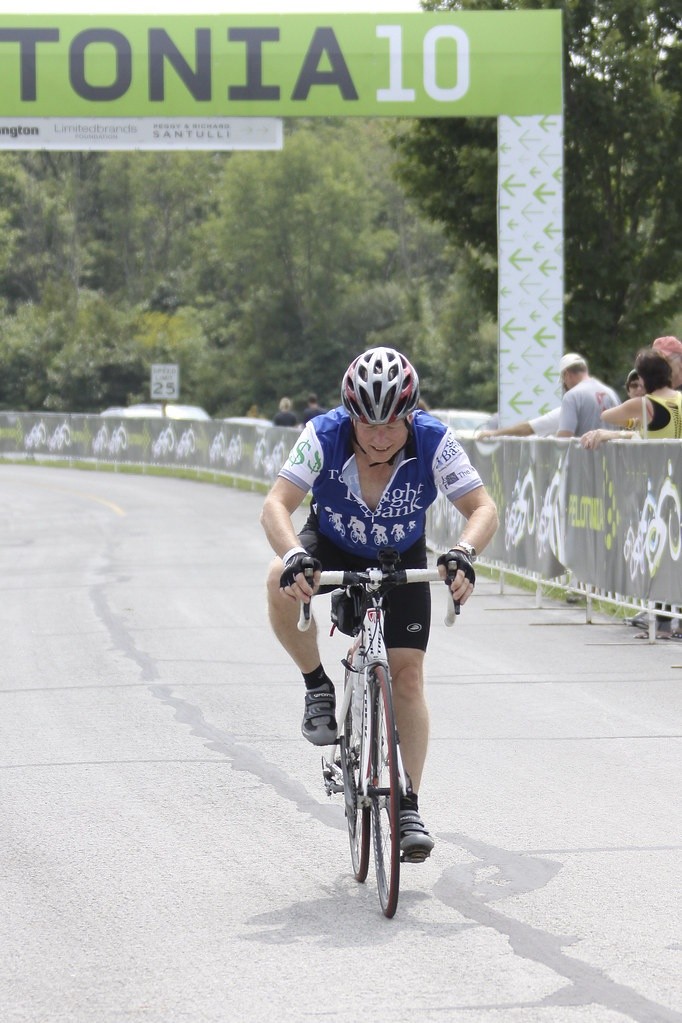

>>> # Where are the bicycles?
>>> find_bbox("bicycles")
[294,556,462,921]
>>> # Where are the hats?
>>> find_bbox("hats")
[653,336,682,360]
[558,353,586,383]
[625,369,637,397]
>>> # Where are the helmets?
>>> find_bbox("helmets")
[341,347,419,426]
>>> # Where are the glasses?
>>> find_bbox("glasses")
[629,383,642,389]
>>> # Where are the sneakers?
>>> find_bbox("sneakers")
[399,800,434,850]
[302,676,338,745]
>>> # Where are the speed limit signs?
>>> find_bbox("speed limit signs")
[152,363,178,401]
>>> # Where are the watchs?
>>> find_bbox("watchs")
[456,541,477,563]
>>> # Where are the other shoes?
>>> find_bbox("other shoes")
[631,611,660,631]
[566,594,583,602]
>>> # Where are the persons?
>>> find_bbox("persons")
[475,335,682,640]
[303,393,326,428]
[272,398,300,428]
[261,346,500,855]
